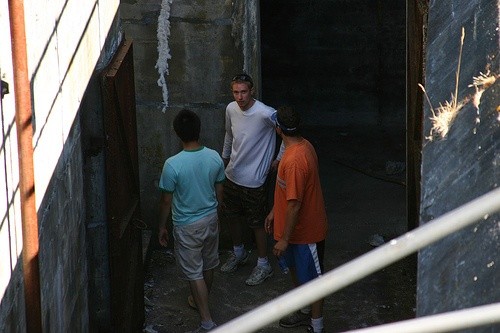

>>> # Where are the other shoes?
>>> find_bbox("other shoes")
[187,295,197,309]
[193,322,219,333]
[304,326,327,333]
[278,309,311,327]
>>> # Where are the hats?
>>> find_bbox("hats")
[271,111,296,132]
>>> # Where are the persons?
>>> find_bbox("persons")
[156,109,225,333]
[263,106,329,333]
[221,74,286,286]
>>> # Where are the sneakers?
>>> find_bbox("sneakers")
[245,263,274,286]
[220,250,249,272]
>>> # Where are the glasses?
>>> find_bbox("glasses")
[232,75,253,86]
